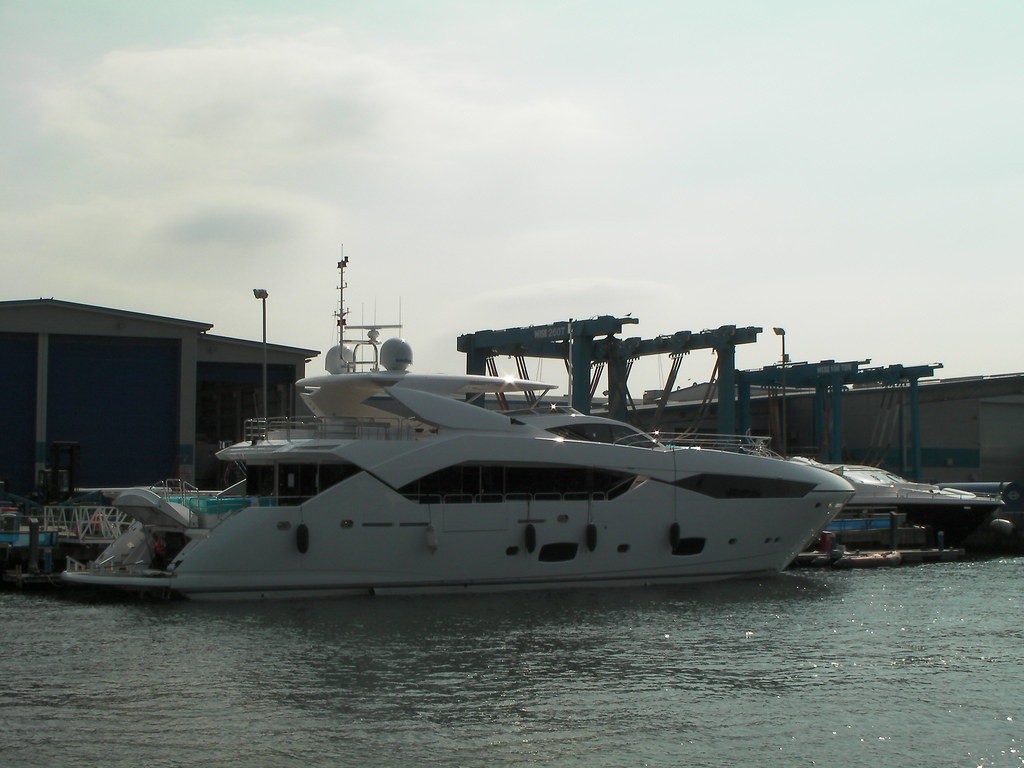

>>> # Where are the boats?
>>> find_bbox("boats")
[60,244,862,600]
[810,547,901,568]
[816,460,1004,545]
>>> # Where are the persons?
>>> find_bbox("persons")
[152,533,168,569]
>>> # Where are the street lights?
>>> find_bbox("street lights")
[774,328,786,459]
[253,287,270,419]
